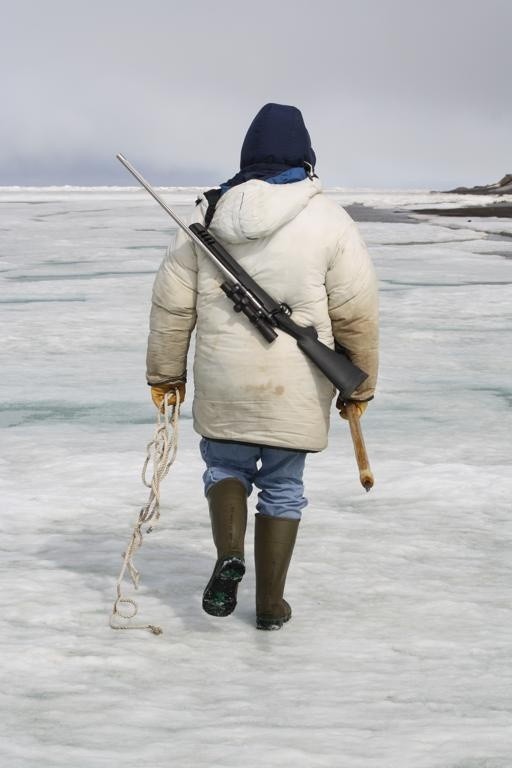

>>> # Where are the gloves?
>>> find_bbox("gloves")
[153,384,186,413]
[341,399,367,422]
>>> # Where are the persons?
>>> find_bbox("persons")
[146,103,380,629]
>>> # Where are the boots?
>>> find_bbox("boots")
[202,478,248,618]
[254,514,301,630]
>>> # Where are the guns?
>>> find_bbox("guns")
[115,154,368,401]
